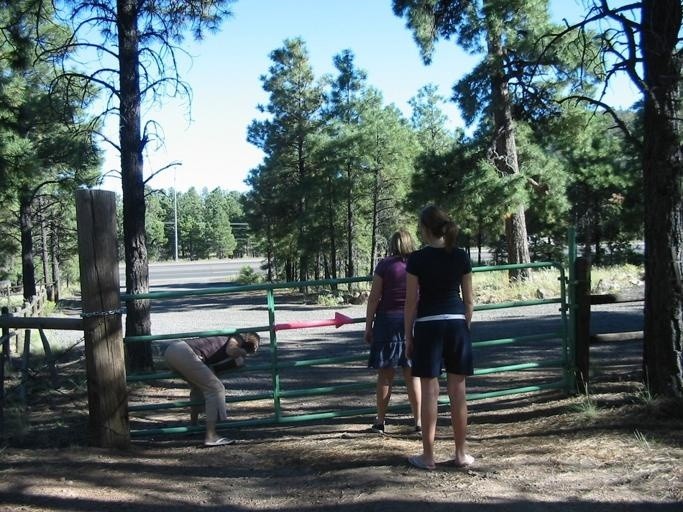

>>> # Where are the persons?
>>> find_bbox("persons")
[162,329,258,447]
[403,205,478,471]
[365,227,424,433]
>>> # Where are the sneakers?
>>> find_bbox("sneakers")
[372,419,385,435]
[414,426,423,433]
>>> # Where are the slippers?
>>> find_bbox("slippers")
[408,455,437,471]
[454,455,474,467]
[204,436,236,447]
